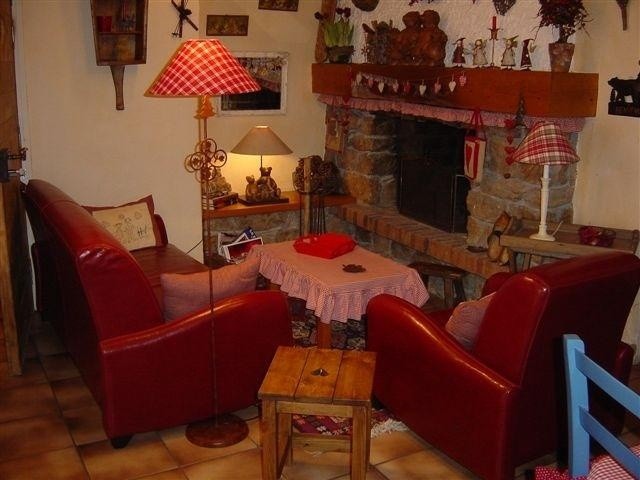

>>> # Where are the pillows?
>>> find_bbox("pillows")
[84,195,167,253]
[446,291,495,344]
[159,254,261,327]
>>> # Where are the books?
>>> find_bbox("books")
[202,191,238,211]
[216,227,264,264]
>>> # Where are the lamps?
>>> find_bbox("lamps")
[144,38,261,447]
[230,126,291,202]
[514,122,580,243]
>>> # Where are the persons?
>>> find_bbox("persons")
[500,35,519,71]
[472,39,488,69]
[452,37,466,68]
[520,38,536,71]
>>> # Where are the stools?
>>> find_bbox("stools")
[258,346,376,480]
[408,262,467,308]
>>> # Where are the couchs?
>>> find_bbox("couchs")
[22,178,288,448]
[367,250,640,480]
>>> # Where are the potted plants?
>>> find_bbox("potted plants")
[532,0,594,72]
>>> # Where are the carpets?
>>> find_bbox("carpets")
[288,309,405,443]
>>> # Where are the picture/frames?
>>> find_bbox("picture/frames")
[207,15,249,36]
[258,0,299,11]
[216,50,288,115]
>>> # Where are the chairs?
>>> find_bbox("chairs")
[562,331,640,480]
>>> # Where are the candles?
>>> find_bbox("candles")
[492,16,497,29]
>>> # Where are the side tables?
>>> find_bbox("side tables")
[502,219,639,275]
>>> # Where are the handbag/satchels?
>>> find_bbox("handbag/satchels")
[463,111,486,182]
[294,232,357,261]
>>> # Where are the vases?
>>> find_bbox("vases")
[328,46,354,62]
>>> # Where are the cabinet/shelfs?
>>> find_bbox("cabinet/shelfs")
[89,0,148,66]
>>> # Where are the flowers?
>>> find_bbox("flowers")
[314,7,355,48]
